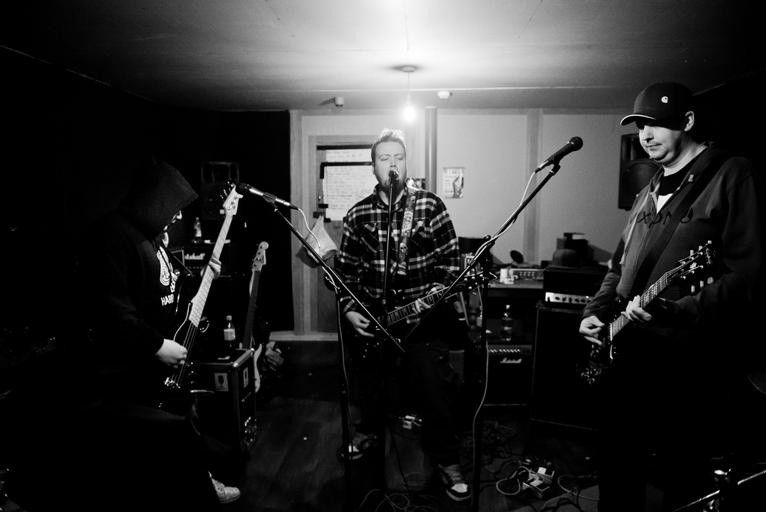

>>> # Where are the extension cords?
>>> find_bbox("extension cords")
[518,466,557,498]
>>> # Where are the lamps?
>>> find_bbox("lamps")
[398,66,422,125]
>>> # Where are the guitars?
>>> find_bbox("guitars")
[239,242,270,392]
[159,180,245,412]
[580,240,721,384]
[348,273,496,379]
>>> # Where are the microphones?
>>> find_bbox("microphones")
[234,182,296,211]
[388,169,399,179]
[533,136,583,174]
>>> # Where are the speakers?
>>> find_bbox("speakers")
[527,307,614,432]
[268,331,339,386]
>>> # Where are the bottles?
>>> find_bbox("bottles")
[221,314,240,357]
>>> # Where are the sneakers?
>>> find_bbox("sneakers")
[338,432,375,460]
[208,473,240,502]
[439,463,471,501]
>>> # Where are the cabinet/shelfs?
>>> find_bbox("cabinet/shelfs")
[193,346,259,476]
[480,264,613,429]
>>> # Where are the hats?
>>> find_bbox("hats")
[621,83,696,125]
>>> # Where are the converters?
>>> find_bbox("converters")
[537,466,556,484]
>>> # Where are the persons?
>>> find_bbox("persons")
[78,160,244,504]
[334,128,471,505]
[577,83,765,512]
